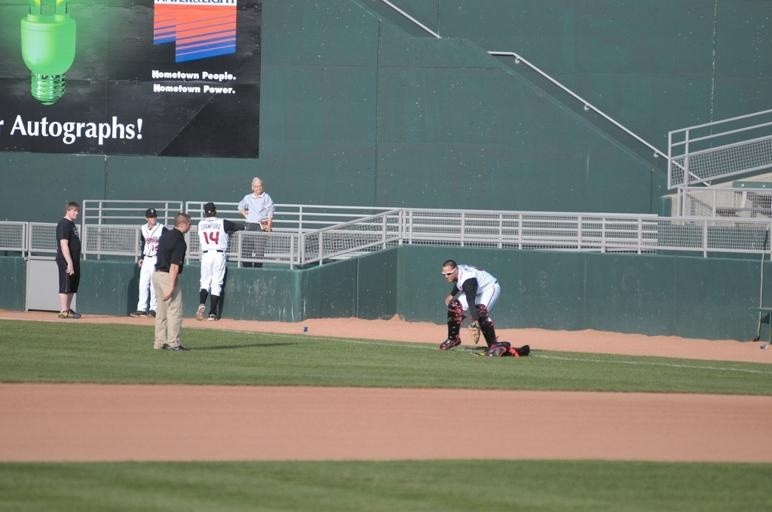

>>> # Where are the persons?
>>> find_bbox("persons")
[152,213,194,353]
[437,258,530,358]
[56,201,82,321]
[128,207,165,319]
[196,201,245,321]
[236,175,276,269]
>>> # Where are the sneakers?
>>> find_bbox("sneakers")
[196,304,205,320]
[440,337,460,349]
[59,309,81,319]
[130,310,155,318]
[154,342,187,352]
[208,313,217,321]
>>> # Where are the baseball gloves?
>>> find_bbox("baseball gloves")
[469,320,480,343]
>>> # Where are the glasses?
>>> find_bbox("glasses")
[441,268,456,276]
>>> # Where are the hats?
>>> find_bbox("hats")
[146,208,156,216]
[205,203,216,212]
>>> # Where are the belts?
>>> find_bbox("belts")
[203,249,223,253]
